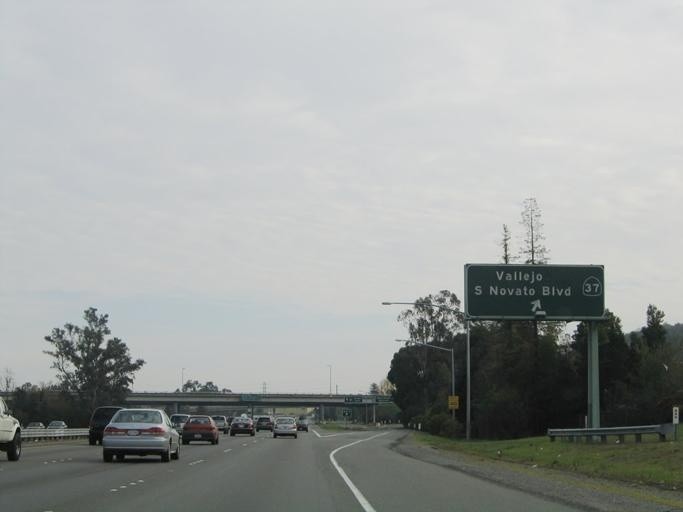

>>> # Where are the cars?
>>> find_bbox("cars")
[89,406,308,462]
[26,421,67,429]
[0,397,21,461]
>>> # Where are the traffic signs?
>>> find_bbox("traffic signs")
[464,265,605,321]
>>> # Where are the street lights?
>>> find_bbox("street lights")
[328,365,332,397]
[381,302,470,440]
[182,367,186,385]
